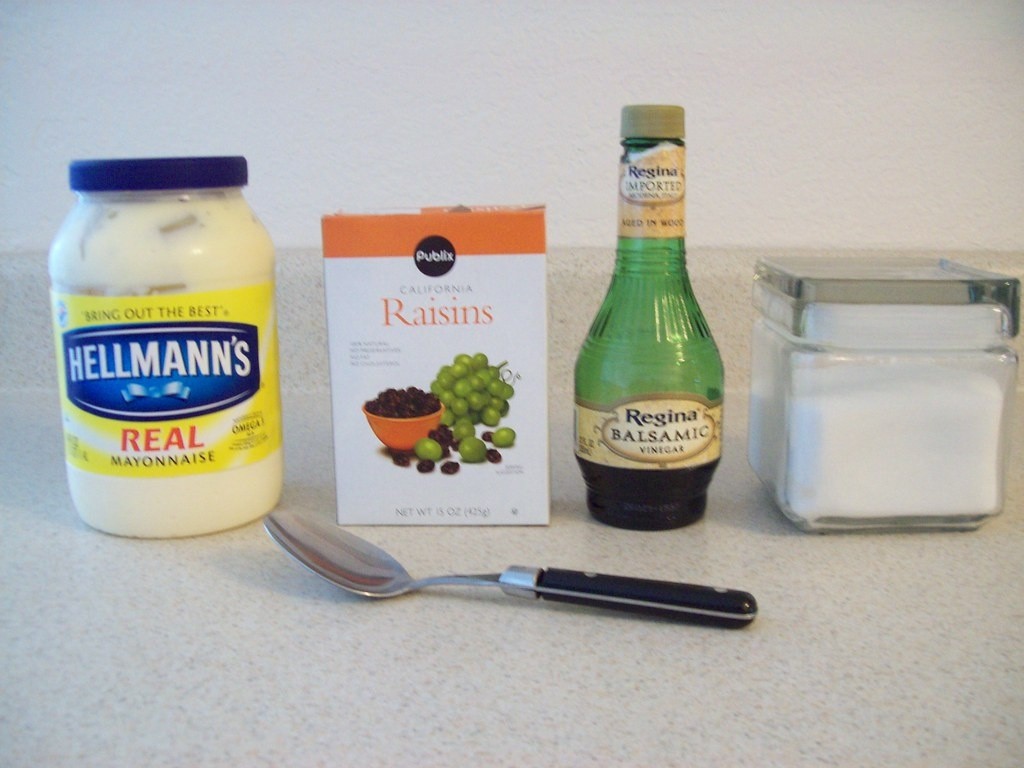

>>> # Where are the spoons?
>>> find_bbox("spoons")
[261,510,758,630]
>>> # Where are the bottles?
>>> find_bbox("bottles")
[575,105,723,531]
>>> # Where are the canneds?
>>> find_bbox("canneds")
[47,153,284,537]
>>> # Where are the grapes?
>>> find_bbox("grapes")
[413,351,517,464]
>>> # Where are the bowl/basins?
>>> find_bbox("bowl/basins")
[362,400,445,450]
